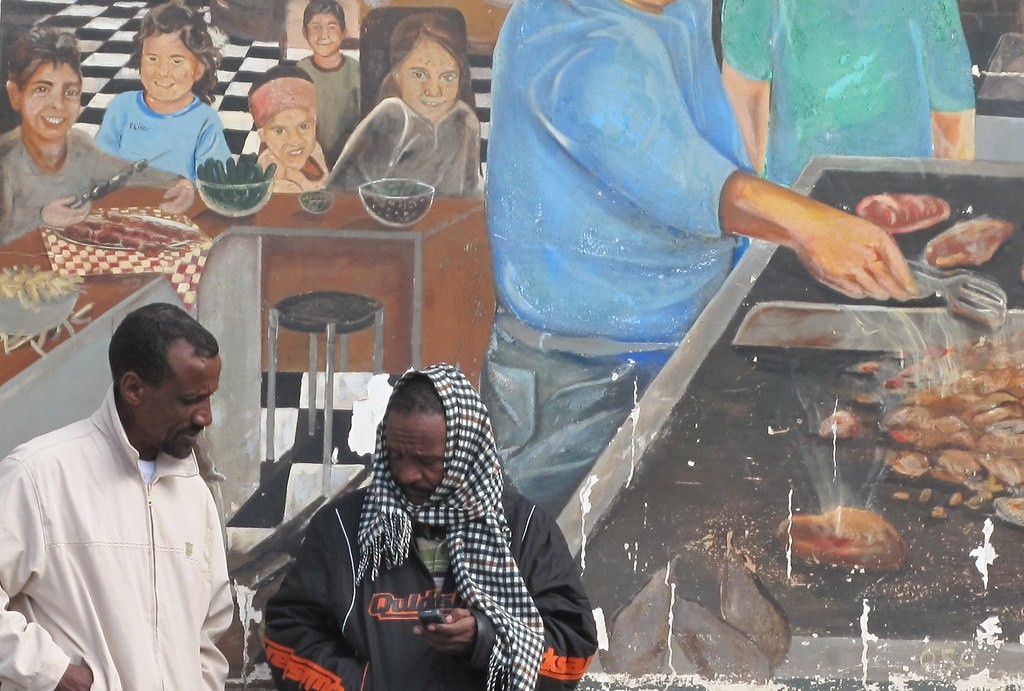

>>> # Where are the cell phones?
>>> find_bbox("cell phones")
[419,609,446,629]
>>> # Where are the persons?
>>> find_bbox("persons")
[264,363,598,691]
[0,302,234,691]
